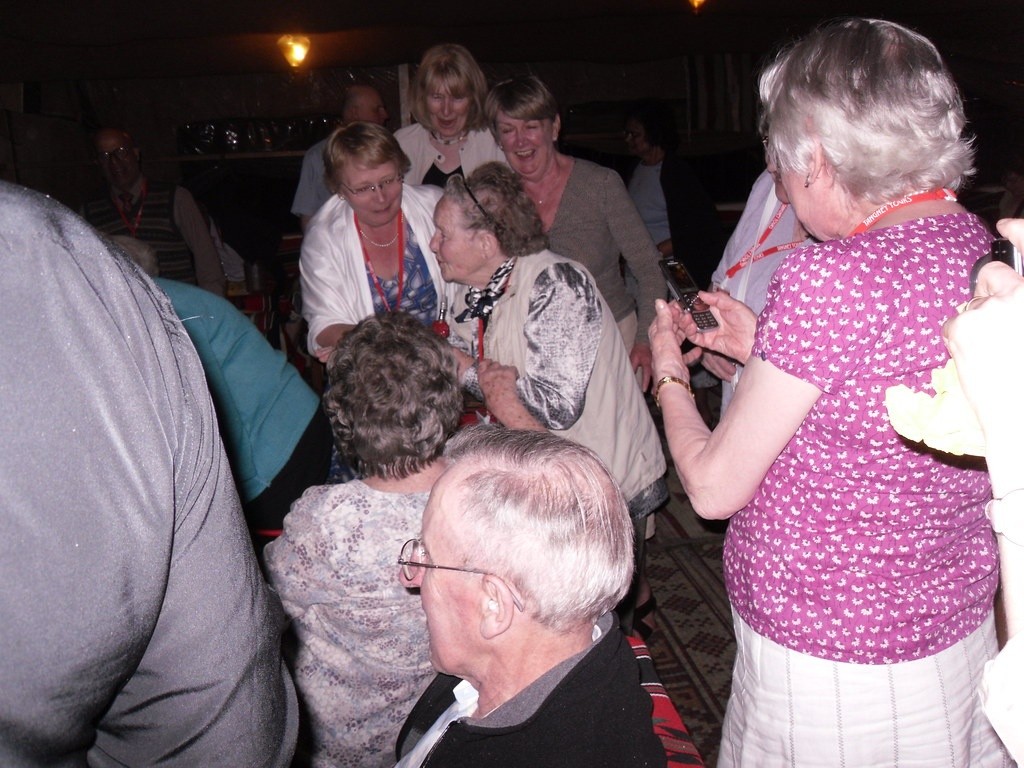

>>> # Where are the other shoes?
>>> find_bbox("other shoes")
[630,596,657,638]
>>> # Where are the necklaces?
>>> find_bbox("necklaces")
[360,213,402,246]
[432,136,463,145]
[539,200,542,204]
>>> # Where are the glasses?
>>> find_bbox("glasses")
[397,538,524,614]
[337,170,402,197]
[96,146,133,167]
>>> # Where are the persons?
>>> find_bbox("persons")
[431,161,689,563]
[941,218,1024,768]
[648,20,1018,768]
[78,128,251,298]
[105,236,334,587]
[0,185,300,768]
[300,122,503,428]
[696,112,820,531]
[262,312,462,768]
[392,429,666,768]
[292,42,721,640]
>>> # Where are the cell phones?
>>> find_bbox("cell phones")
[990,238,1022,277]
[658,258,721,333]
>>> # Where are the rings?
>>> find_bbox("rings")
[966,295,982,310]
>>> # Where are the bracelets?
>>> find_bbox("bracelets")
[656,376,690,407]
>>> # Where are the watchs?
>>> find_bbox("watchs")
[985,489,1024,548]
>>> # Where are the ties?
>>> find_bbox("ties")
[119,191,134,214]
